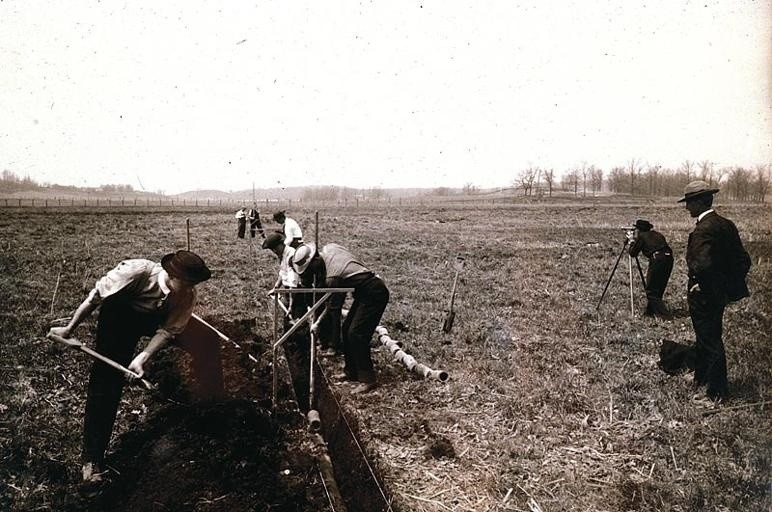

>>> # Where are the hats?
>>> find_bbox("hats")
[161,250,211,281]
[633,220,653,228]
[262,233,287,248]
[677,181,720,202]
[272,209,287,221]
[292,241,317,275]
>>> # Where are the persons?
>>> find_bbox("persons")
[234,205,246,239]
[49,248,226,484]
[675,179,752,400]
[627,218,673,321]
[248,201,266,240]
[261,209,391,384]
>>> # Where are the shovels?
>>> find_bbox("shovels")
[443,257,465,332]
[45,331,193,408]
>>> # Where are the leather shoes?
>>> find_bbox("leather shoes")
[350,382,378,395]
[334,373,351,382]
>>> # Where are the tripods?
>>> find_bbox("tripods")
[595,236,648,316]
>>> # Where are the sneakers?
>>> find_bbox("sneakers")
[81,461,108,496]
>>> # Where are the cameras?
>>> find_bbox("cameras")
[619,227,636,236]
